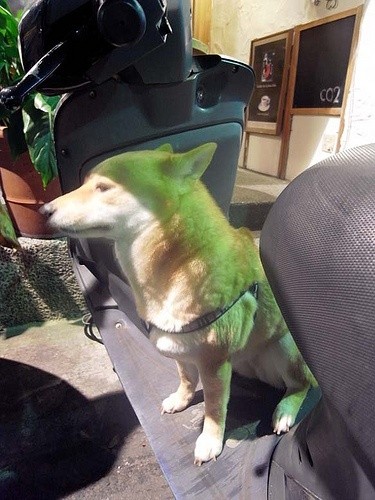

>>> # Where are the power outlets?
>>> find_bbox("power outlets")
[321,132,337,154]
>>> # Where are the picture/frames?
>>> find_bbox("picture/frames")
[243,28,293,136]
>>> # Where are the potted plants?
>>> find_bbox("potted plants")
[0,0,68,240]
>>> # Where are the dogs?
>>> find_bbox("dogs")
[39,141,320,466]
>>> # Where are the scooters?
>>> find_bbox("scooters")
[1,0,375,500]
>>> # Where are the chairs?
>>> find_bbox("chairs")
[18,0,257,267]
